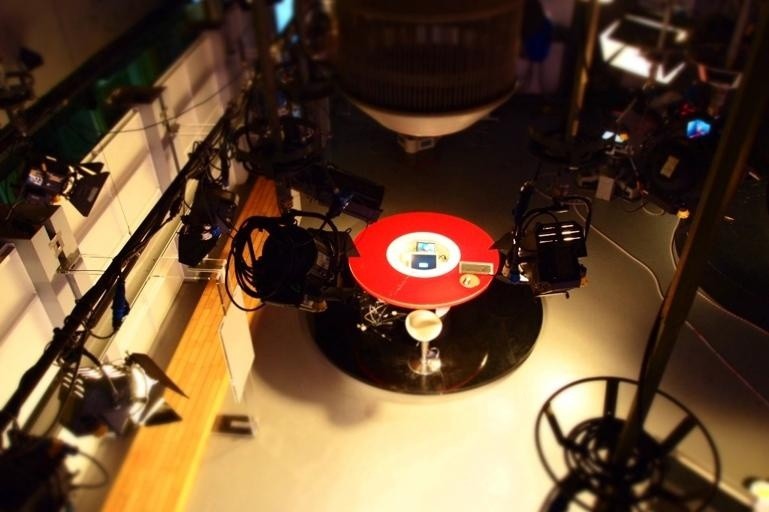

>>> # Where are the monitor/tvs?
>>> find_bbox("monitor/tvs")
[272,0,296,36]
[683,117,713,139]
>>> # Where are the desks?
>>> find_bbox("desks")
[347,210,501,311]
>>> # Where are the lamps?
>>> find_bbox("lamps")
[332,1,523,138]
[0,430,108,512]
[225,209,355,313]
[27,169,111,218]
[57,347,137,437]
[177,223,220,266]
[598,13,688,85]
[506,184,593,300]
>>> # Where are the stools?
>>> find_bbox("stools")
[404,309,443,376]
